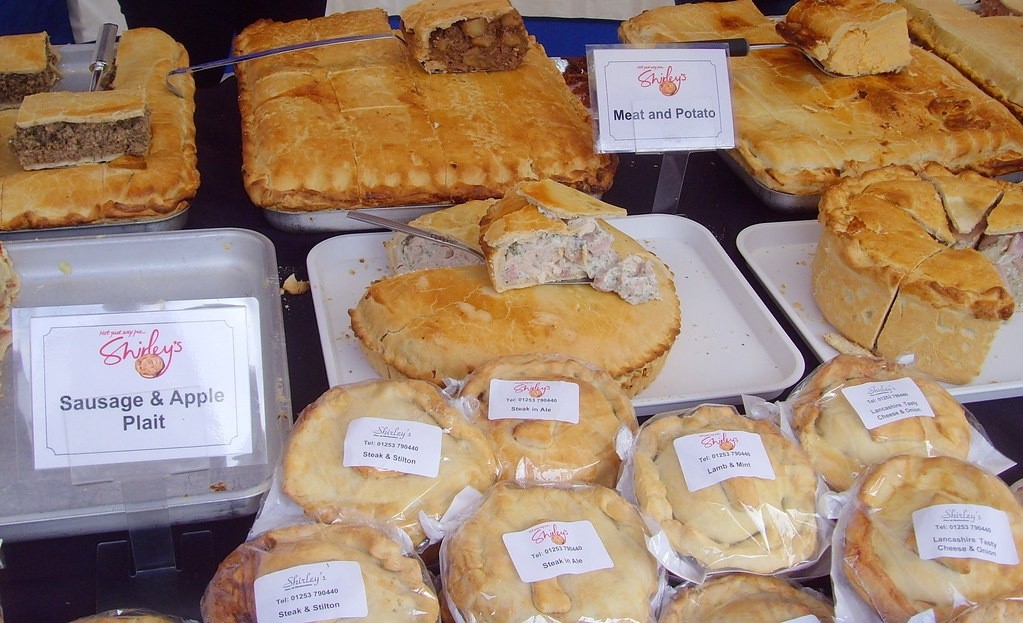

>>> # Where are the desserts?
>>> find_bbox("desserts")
[60,355,1023,623]
[0,0,1023,405]
